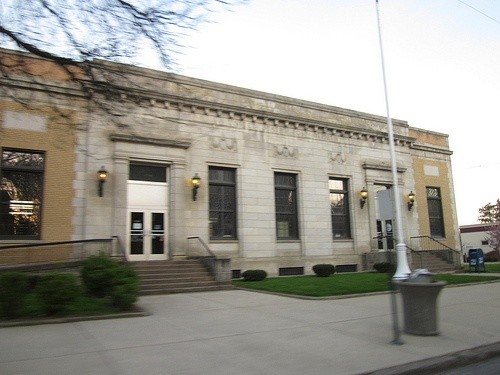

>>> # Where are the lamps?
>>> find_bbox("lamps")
[360,186,368,209]
[408,191,416,210]
[98,166,108,198]
[191,172,201,200]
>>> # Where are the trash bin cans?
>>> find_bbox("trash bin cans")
[466,248,485,267]
